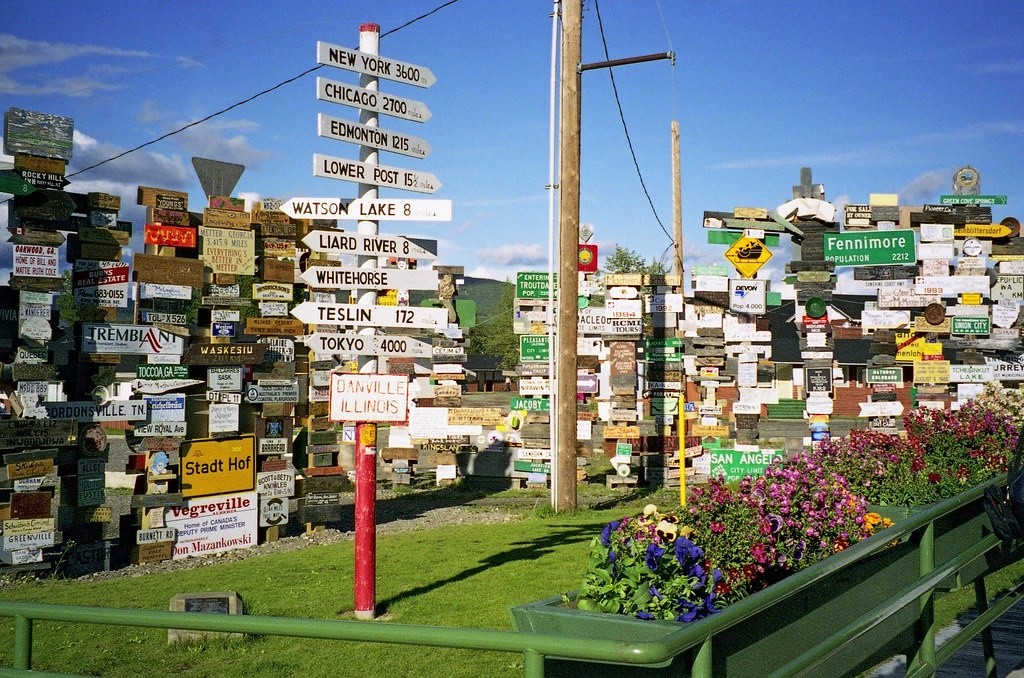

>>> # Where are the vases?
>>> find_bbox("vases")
[512,486,1024,678]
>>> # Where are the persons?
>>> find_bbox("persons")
[504,377,512,392]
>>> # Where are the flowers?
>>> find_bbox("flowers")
[593,386,1024,615]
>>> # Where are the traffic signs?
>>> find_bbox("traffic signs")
[275,41,452,354]
